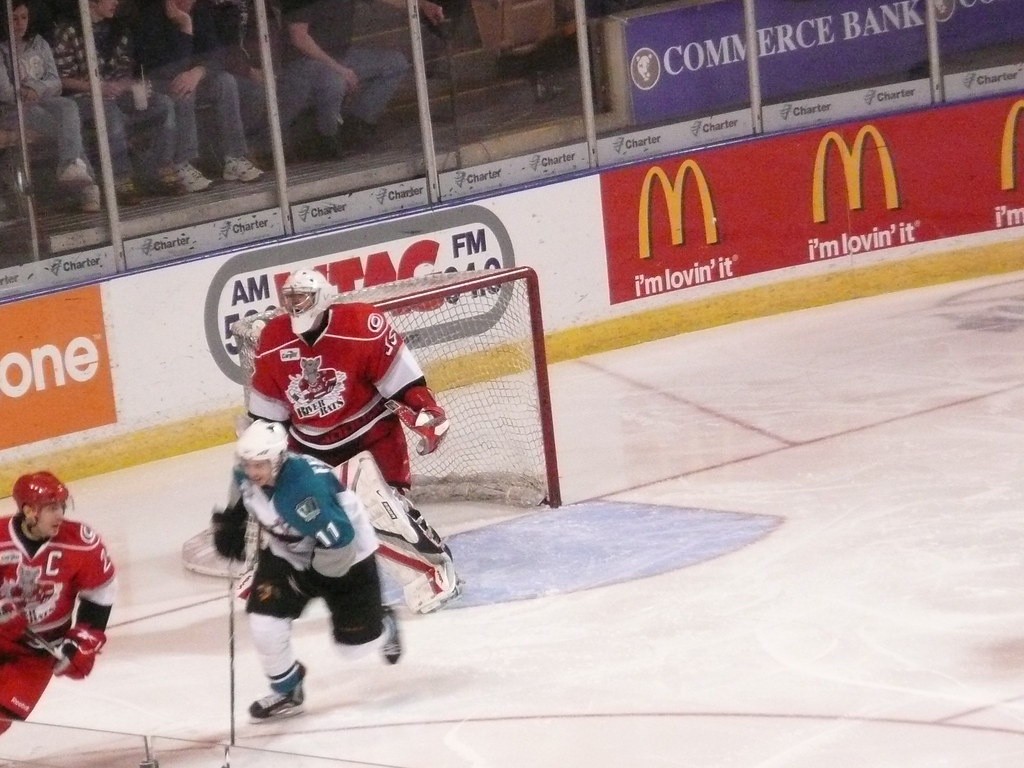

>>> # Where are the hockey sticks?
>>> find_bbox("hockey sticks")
[225,552,236,754]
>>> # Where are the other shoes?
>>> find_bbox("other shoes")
[320,135,348,158]
[149,168,181,195]
[350,132,390,153]
[115,178,140,206]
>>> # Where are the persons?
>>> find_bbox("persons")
[246,269,463,616]
[209,418,403,724]
[0,470,115,733]
[0,0,444,212]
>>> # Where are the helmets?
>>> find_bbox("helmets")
[11,470,76,514]
[279,268,338,335]
[237,418,288,477]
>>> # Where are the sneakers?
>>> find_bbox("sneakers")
[58,158,91,183]
[222,154,264,182]
[378,606,402,664]
[174,164,214,193]
[248,679,306,725]
[80,184,101,212]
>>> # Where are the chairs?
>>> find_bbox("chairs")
[0,1,595,213]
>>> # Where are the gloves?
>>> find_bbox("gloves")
[302,565,337,598]
[50,622,107,680]
[213,509,248,557]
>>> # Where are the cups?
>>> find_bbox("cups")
[133,82,148,112]
